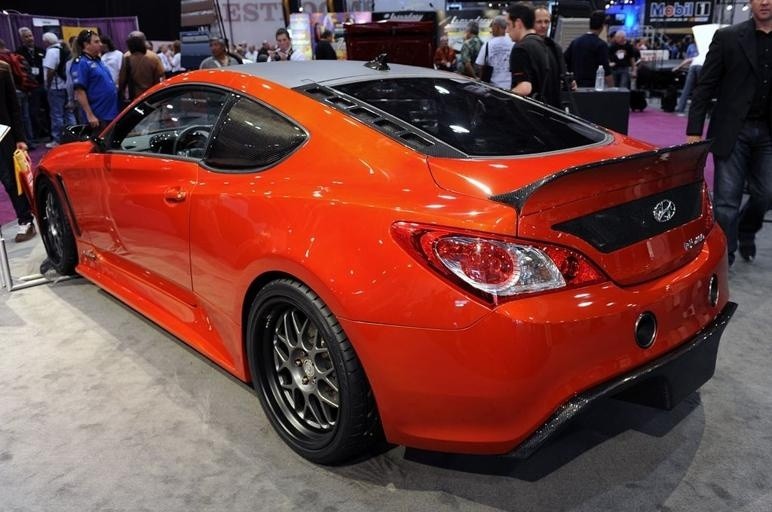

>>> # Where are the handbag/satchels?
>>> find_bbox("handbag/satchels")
[59,121,103,143]
[118,72,134,103]
[482,65,493,83]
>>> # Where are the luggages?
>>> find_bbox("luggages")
[662,84,676,112]
[630,89,646,112]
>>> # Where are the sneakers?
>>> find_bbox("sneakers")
[45,141,60,148]
[15,218,36,242]
[739,233,756,261]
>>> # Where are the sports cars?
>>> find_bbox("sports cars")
[32,52,740,483]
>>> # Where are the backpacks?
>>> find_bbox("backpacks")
[9,52,40,90]
[55,42,73,81]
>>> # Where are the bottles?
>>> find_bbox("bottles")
[595,64,605,91]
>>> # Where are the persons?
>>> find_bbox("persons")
[434,3,702,113]
[684,0,772,266]
[199,29,307,69]
[315,30,337,60]
[0,27,187,243]
